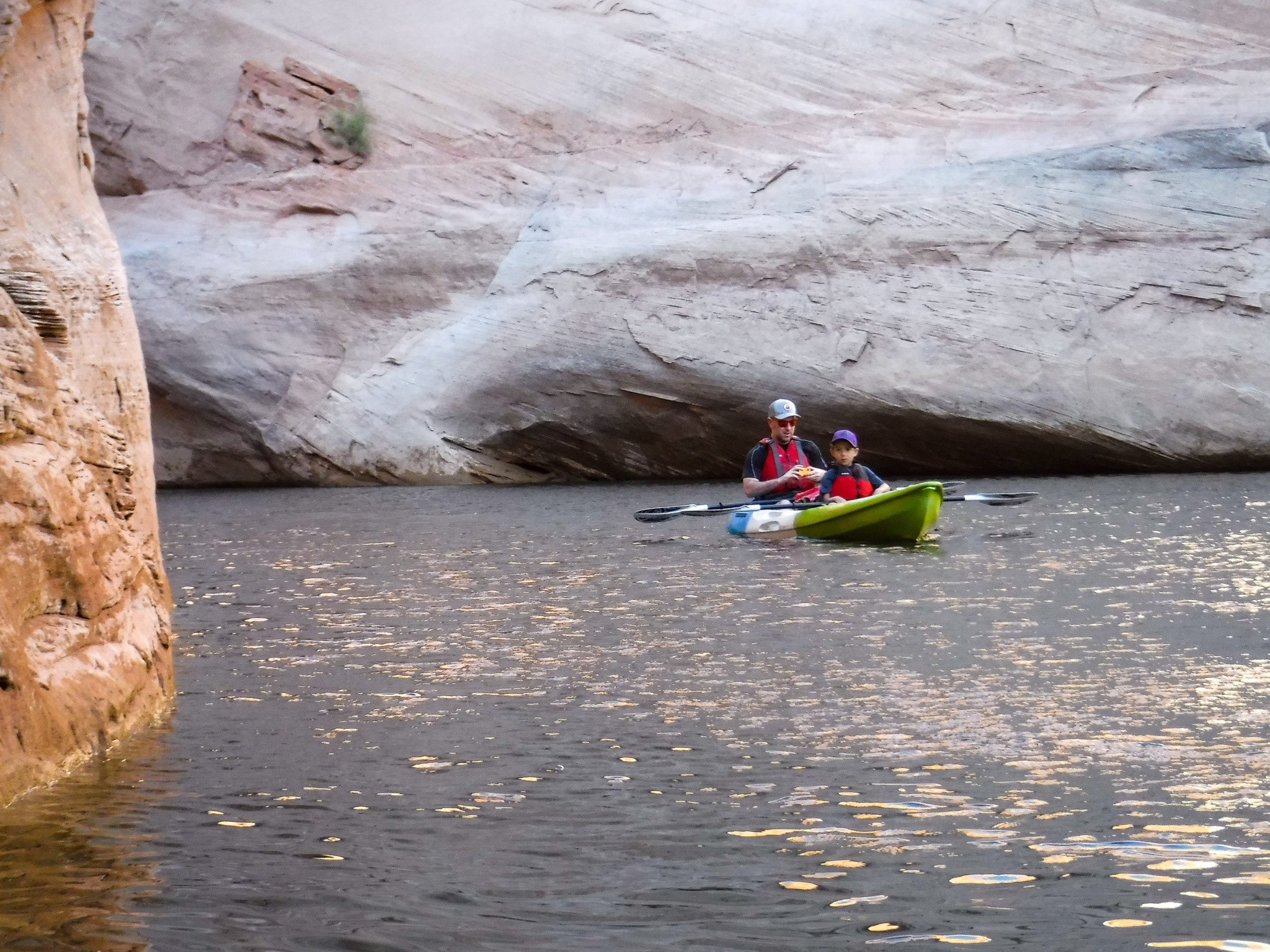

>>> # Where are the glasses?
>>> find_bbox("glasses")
[771,417,799,427]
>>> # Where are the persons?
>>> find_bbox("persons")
[820,430,891,506]
[741,398,827,498]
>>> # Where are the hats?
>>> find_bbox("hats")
[768,399,802,420]
[831,430,857,448]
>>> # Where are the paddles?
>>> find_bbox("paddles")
[634,481,1039,524]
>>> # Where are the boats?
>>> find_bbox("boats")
[727,480,944,543]
